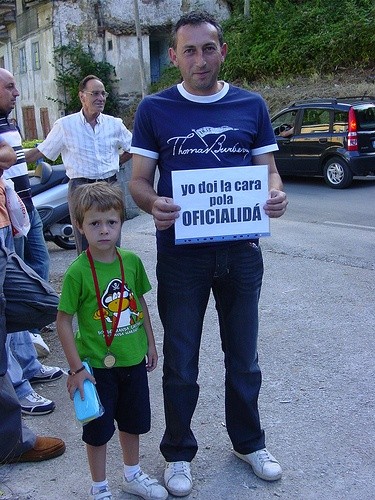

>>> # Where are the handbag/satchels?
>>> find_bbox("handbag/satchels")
[3,178,30,239]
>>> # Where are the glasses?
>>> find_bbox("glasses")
[84,91,109,98]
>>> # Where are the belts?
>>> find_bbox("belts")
[79,173,117,184]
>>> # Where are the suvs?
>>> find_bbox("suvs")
[268,95,375,188]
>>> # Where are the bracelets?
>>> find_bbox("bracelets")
[68,366,85,375]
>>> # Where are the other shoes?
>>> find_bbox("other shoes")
[0,436,66,463]
[28,331,50,357]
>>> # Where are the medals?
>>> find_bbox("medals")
[104,354,116,368]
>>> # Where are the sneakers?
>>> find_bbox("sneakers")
[18,390,54,415]
[233,447,282,481]
[164,460,193,497]
[88,485,114,500]
[122,470,168,500]
[28,364,63,385]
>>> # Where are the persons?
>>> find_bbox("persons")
[55,182,168,500]
[0,135,66,464]
[127,12,288,497]
[0,68,50,357]
[25,74,133,256]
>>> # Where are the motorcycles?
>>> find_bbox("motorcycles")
[27,160,78,249]
